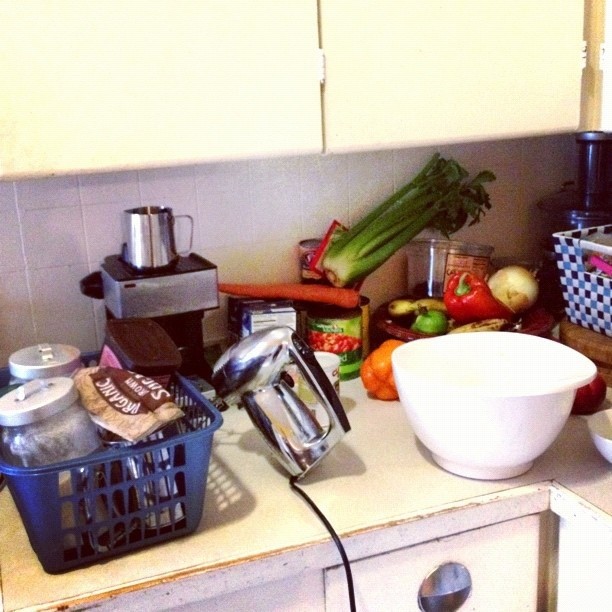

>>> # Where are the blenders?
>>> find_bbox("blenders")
[188,324,353,482]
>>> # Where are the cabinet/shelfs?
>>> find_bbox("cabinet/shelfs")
[0,1,587,182]
[0,484,612,611]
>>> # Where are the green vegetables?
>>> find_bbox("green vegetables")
[323,151,497,288]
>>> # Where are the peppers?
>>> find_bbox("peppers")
[444,271,516,326]
[360,338,407,401]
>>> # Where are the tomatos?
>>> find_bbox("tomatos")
[571,373,607,415]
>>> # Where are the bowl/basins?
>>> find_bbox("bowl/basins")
[390,330,598,481]
[587,408,611,464]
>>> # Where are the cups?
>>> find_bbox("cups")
[314,351,339,398]
[123,206,194,270]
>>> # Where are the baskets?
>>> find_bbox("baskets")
[552,224,612,339]
[1,350,224,574]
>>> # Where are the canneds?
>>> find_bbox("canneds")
[299,238,323,285]
[297,294,370,382]
[299,350,341,405]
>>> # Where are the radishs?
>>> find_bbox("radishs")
[487,264,539,315]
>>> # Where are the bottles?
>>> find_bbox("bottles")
[360,296,370,358]
[306,309,363,383]
[7,342,85,387]
[0,379,109,551]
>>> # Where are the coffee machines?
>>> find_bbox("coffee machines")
[540,132,611,287]
